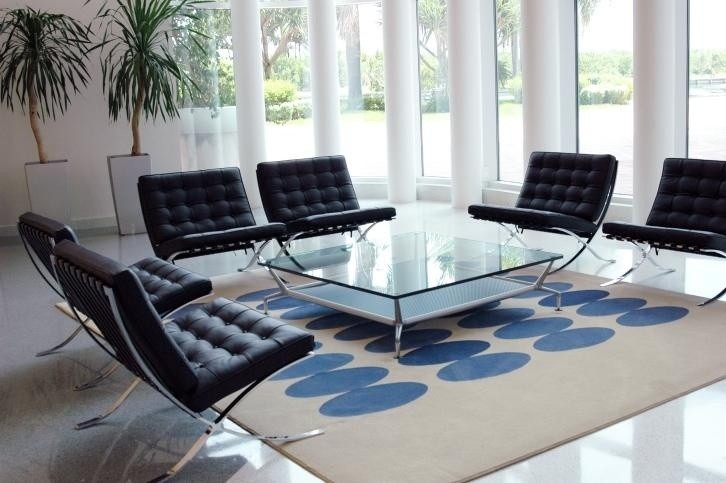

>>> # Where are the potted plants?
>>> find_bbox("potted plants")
[77,2,219,236]
[0,1,95,237]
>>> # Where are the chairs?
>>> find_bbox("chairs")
[264,154,399,271]
[12,210,215,391]
[133,165,290,286]
[599,157,725,306]
[468,153,618,274]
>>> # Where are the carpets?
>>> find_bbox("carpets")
[50,266,726,483]
